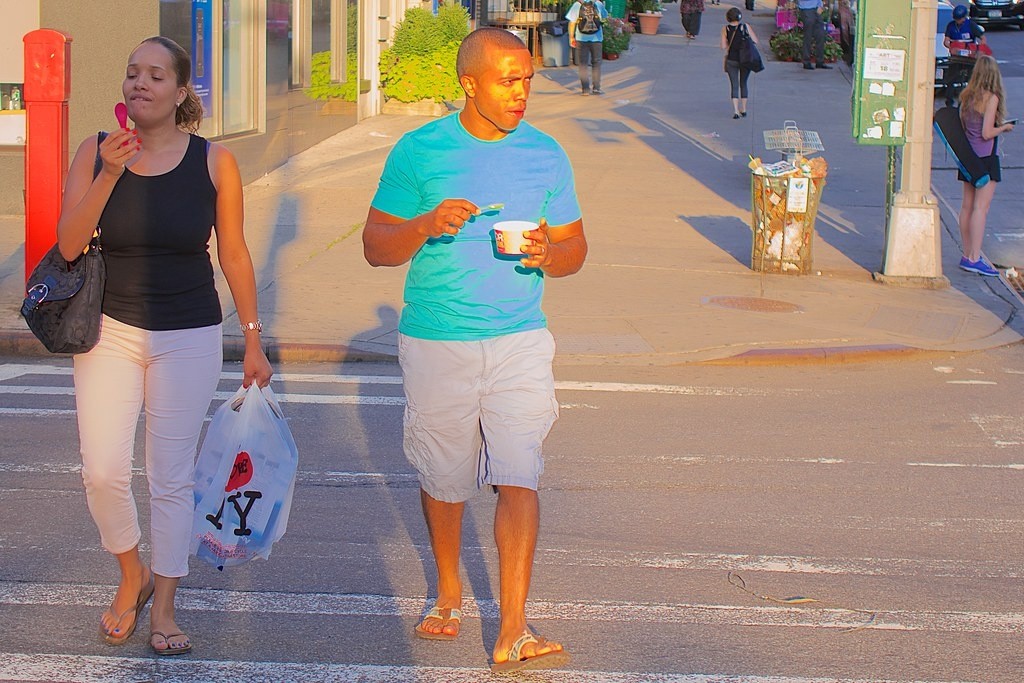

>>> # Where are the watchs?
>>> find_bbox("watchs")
[240,319,263,332]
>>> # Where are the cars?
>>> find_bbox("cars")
[967,0,1024,31]
[934,2,986,89]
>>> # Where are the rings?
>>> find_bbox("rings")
[541,247,545,255]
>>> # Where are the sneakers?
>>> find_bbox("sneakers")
[959,256,965,268]
[964,256,1000,277]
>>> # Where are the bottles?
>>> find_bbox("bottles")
[11,87,21,110]
[1,94,9,109]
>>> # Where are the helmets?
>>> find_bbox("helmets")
[953,4,968,18]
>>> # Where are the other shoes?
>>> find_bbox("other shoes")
[686,32,690,38]
[741,112,746,117]
[717,3,720,5]
[581,88,590,96]
[803,63,815,70]
[593,89,606,95]
[690,34,696,39]
[733,114,739,119]
[816,63,833,69]
[712,2,715,4]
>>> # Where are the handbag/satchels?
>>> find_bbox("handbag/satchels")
[189,379,298,569]
[932,107,990,189]
[21,130,105,354]
[723,55,729,72]
[742,24,765,72]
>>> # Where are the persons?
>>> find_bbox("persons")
[799,0,833,70]
[943,5,986,105]
[712,0,720,5]
[680,0,706,40]
[957,57,1015,276]
[722,7,758,119]
[57,36,273,654]
[363,27,588,671]
[566,0,608,95]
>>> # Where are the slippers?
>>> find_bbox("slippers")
[98,576,155,645]
[490,633,572,672]
[415,607,462,641]
[149,630,191,654]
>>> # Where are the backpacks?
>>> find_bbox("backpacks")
[578,0,600,34]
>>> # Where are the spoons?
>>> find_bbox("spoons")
[114,102,130,155]
[467,203,504,215]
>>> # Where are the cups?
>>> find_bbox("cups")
[494,221,539,254]
[9,100,18,110]
[748,157,767,182]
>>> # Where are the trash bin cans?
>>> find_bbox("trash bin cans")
[540,21,571,66]
[751,173,825,275]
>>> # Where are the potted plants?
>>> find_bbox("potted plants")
[600,0,666,61]
[769,24,843,64]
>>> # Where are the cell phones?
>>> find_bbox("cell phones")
[1005,120,1018,125]
